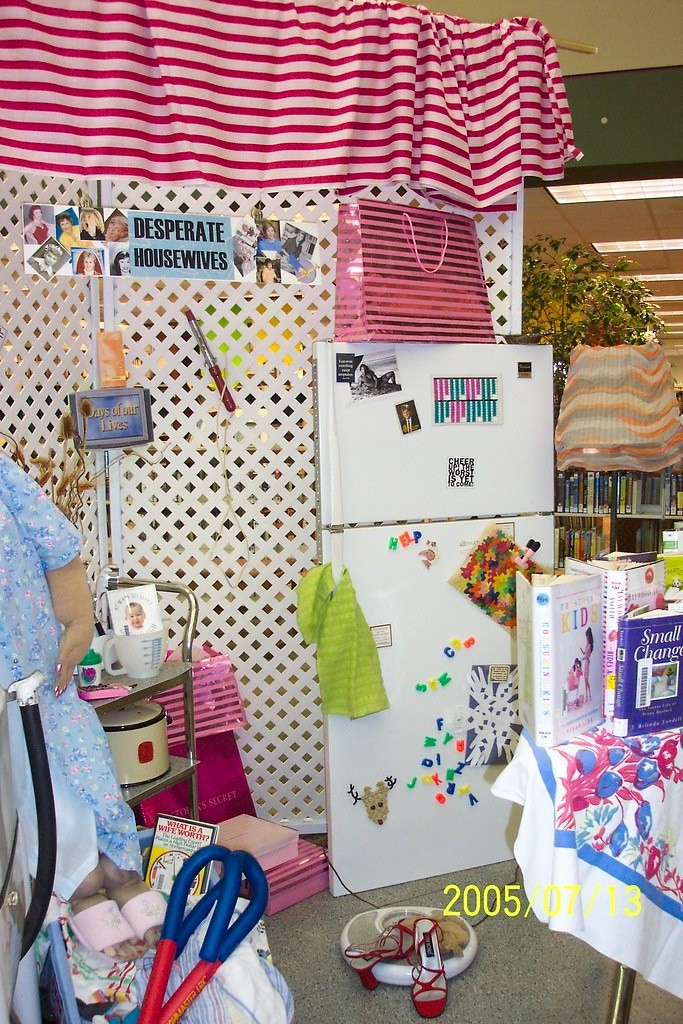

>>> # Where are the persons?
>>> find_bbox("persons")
[579,627,594,702]
[105,216,129,241]
[235,221,306,282]
[401,404,417,433]
[123,602,149,635]
[81,209,105,240]
[0,456,173,961]
[112,251,130,276]
[24,205,54,245]
[573,658,583,677]
[78,252,101,275]
[28,244,63,282]
[351,364,401,391]
[57,212,80,253]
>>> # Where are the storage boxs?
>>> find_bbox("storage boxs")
[240,838,328,917]
[213,814,299,881]
[43,827,220,1024]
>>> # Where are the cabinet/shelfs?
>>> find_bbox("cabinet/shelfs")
[74,568,199,823]
[553,465,683,576]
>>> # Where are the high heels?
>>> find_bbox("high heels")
[344,917,417,990]
[407,918,448,1019]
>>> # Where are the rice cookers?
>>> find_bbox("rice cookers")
[92,699,172,787]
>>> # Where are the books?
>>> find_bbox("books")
[554,469,683,568]
[516,552,683,749]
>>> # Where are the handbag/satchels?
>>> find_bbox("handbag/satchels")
[334,197,496,344]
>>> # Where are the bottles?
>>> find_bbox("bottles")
[77,649,103,687]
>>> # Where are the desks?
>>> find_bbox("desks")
[490,728,683,1024]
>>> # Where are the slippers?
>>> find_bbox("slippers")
[68,899,146,960]
[120,890,168,953]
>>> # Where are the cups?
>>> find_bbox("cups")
[103,624,169,679]
